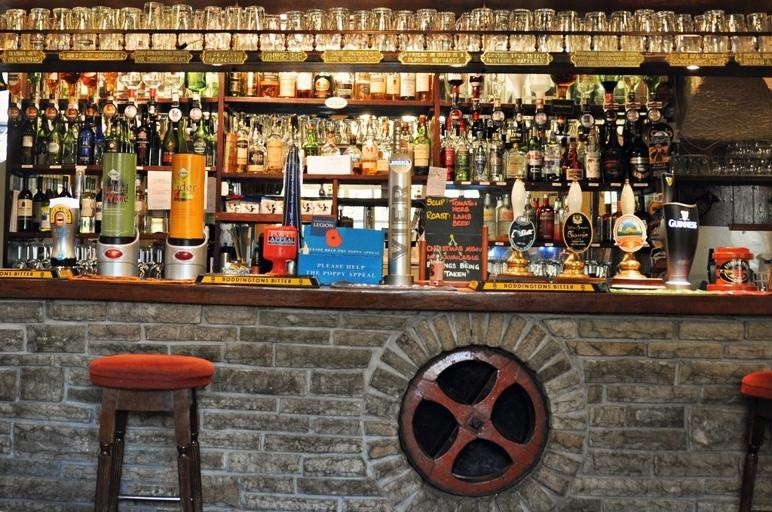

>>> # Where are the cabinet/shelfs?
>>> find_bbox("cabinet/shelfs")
[5,72,665,288]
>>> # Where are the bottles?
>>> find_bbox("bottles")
[706,247,716,285]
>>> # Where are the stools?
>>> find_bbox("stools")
[740,372,772,511]
[89,354,214,512]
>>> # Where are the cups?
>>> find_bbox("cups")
[753,272,769,292]
[672,137,771,176]
